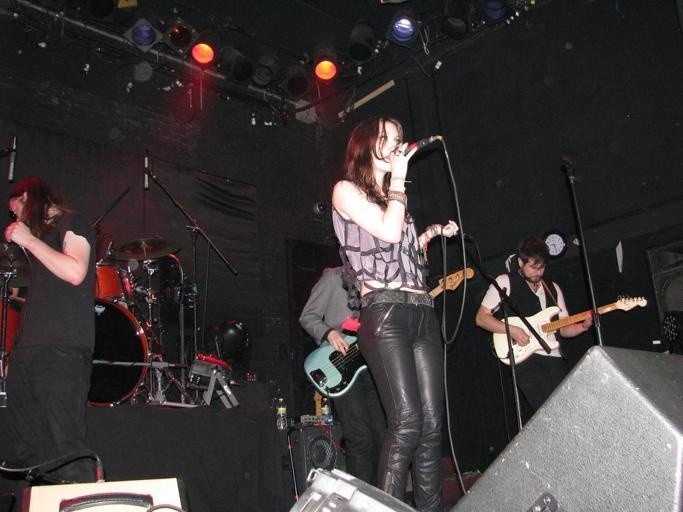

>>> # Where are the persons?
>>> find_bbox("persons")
[0,176,97,484]
[475,236,593,414]
[331,116,459,512]
[299,266,387,485]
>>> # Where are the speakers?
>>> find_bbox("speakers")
[285,425,345,504]
[86,405,292,512]
[449,345,683,512]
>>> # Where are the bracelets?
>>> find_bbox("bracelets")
[423,224,438,241]
[388,191,408,207]
[390,176,406,182]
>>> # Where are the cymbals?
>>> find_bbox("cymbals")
[0,276,31,288]
[104,237,182,260]
[1,250,28,267]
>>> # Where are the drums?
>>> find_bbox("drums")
[90,298,149,407]
[122,254,186,304]
[1,296,28,379]
[95,261,122,300]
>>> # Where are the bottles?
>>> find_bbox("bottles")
[319,398,331,425]
[275,397,288,430]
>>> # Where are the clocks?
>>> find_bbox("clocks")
[541,230,567,259]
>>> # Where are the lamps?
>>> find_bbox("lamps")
[118,0,514,127]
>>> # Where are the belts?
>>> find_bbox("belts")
[360,291,435,308]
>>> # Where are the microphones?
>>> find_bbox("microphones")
[404,135,434,155]
[8,138,17,180]
[144,148,150,189]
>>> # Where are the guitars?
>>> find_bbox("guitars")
[493,294,648,365]
[304,267,473,398]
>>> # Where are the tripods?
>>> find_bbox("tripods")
[107,230,216,407]
